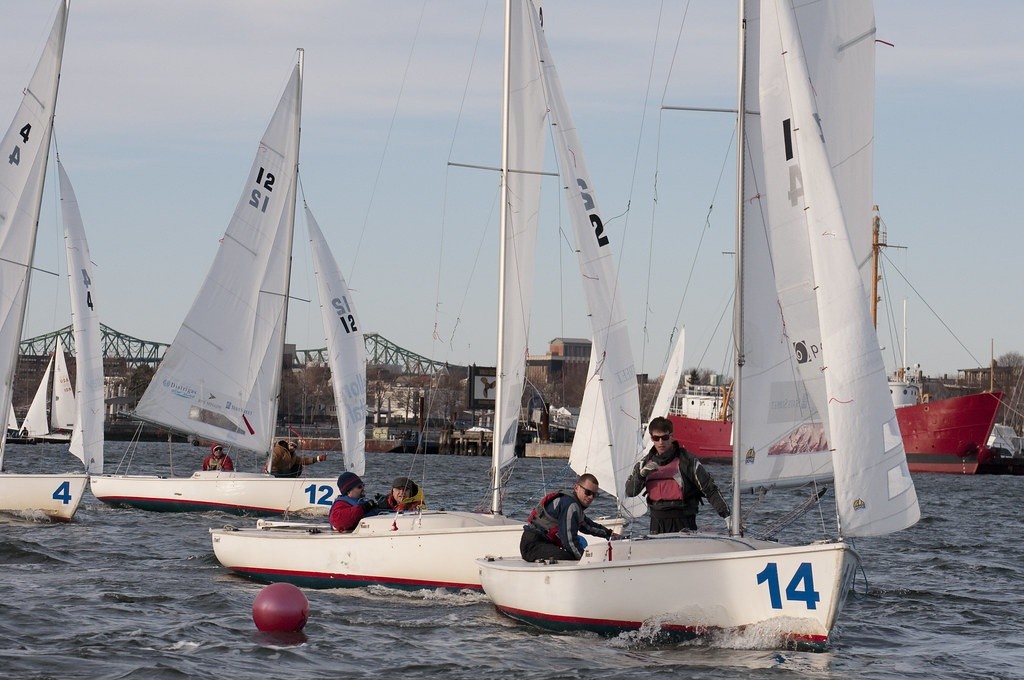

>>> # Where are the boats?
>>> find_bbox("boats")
[668,200,1007,478]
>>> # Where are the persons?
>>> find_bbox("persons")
[328,471,427,534]
[202,443,234,471]
[625,416,747,540]
[264,439,327,475]
[519,472,623,563]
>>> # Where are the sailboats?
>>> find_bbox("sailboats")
[6,400,33,445]
[86,44,367,520]
[0,0,107,521]
[208,3,627,594]
[16,334,76,443]
[476,3,923,650]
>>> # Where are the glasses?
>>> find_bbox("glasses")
[579,484,600,498]
[215,447,222,451]
[352,484,365,489]
[290,443,297,448]
[652,432,672,441]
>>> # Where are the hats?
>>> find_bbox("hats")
[337,472,363,496]
[288,439,298,448]
[392,477,415,491]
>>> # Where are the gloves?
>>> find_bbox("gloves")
[320,454,327,461]
[639,462,658,478]
[363,500,374,511]
[724,516,731,530]
